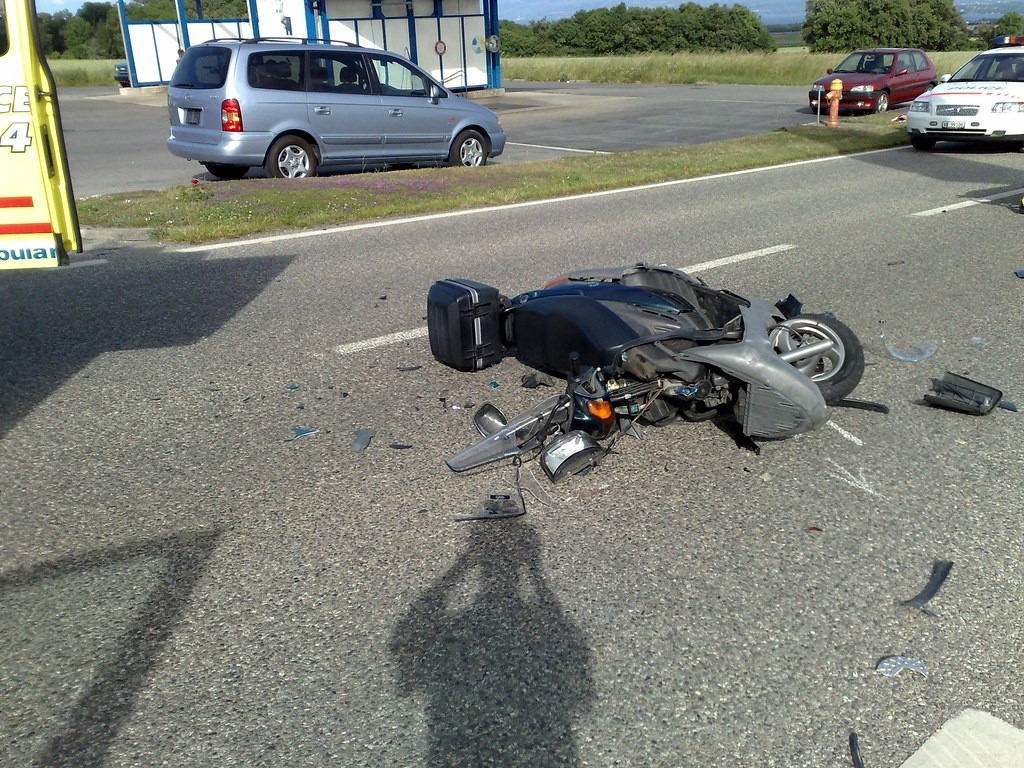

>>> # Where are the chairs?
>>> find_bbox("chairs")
[866,59,878,69]
[252,60,362,92]
[993,60,1024,78]
[898,61,905,69]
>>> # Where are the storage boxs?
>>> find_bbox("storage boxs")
[427,279,503,372]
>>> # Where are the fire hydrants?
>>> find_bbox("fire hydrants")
[824,77,843,129]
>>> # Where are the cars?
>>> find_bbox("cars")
[906,35,1024,151]
[808,48,939,114]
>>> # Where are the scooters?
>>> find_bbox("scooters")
[425,262,890,483]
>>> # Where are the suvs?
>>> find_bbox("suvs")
[165,36,508,179]
